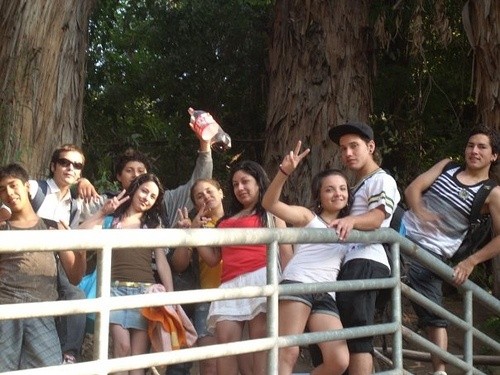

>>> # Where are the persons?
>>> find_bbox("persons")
[328,123,401,375]
[76,115,213,299]
[262,139,355,375]
[174,179,254,375]
[397,127,500,375]
[0,144,87,365]
[0,164,87,372]
[177,160,294,375]
[77,173,198,375]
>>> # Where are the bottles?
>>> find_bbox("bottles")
[187,107,232,154]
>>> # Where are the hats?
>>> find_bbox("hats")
[329,122,374,145]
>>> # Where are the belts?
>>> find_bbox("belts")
[113,281,149,288]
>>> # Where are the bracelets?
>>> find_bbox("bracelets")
[197,148,211,154]
[278,164,290,177]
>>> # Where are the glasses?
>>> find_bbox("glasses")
[57,158,83,169]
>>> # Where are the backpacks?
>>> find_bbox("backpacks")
[442,161,498,263]
